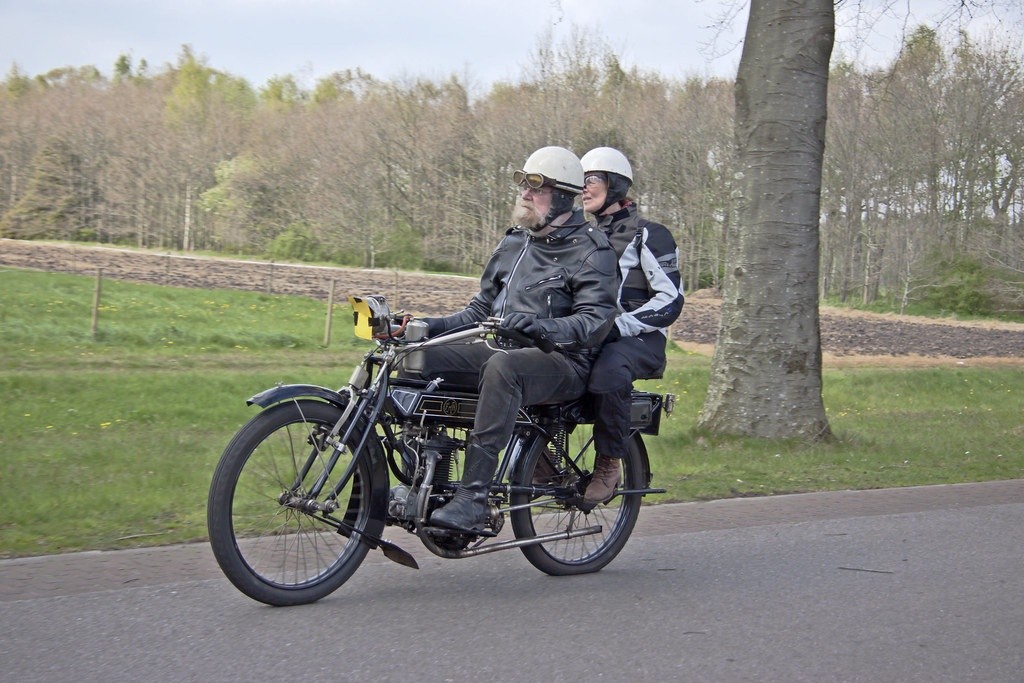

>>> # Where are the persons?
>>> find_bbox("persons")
[516,146,684,505]
[391,147,620,531]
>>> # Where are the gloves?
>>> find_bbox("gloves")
[415,316,445,338]
[501,313,558,354]
[602,322,619,345]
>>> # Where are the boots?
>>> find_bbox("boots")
[430,444,499,530]
[389,442,418,502]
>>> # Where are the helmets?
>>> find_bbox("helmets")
[521,146,585,194]
[580,147,633,187]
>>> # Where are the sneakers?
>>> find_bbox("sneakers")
[583,452,622,503]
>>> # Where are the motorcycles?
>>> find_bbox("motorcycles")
[208,289,675,605]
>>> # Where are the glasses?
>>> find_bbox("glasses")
[513,170,583,194]
[584,174,607,186]
